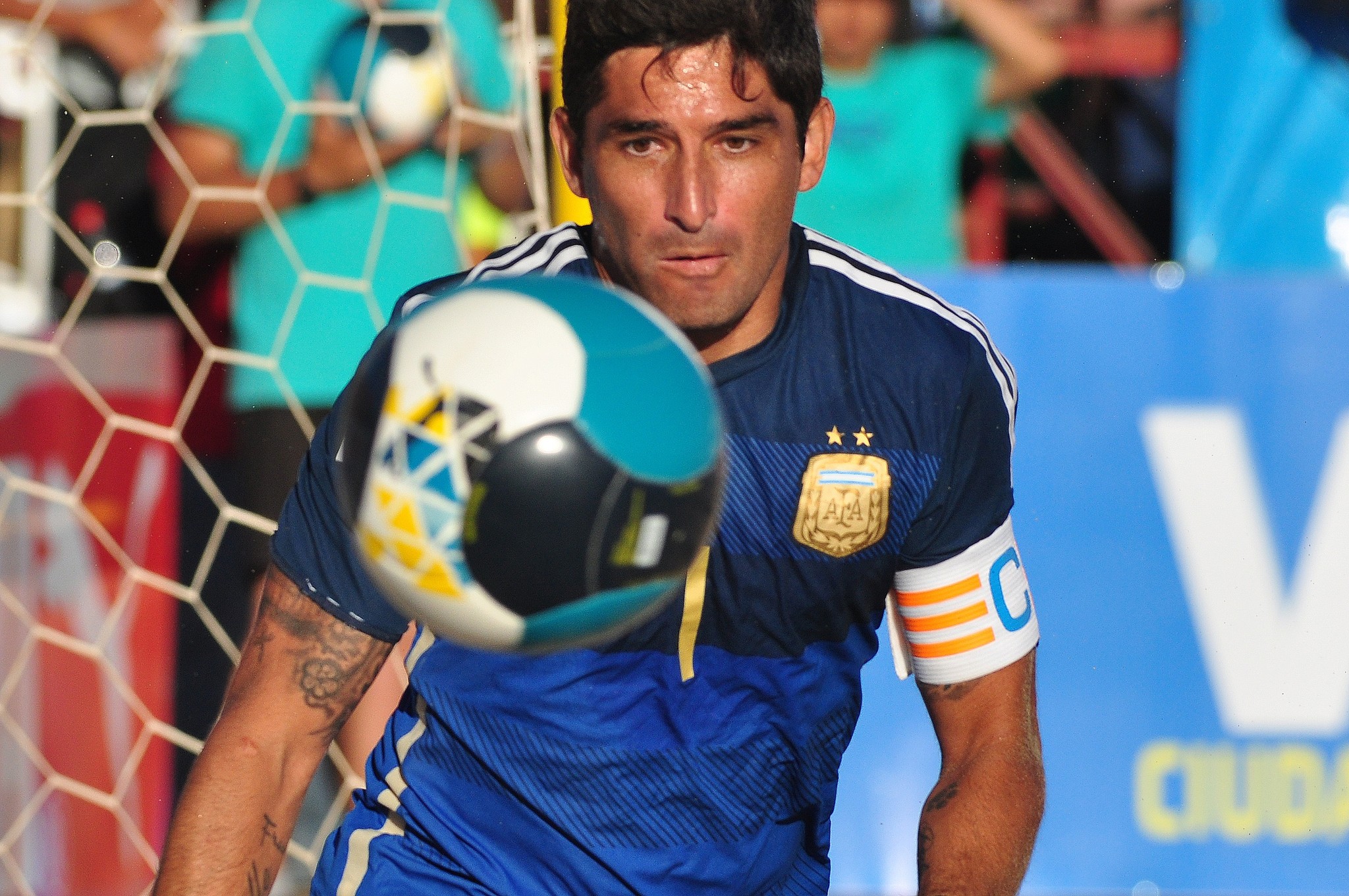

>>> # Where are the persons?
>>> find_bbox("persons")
[0,0,556,896]
[781,0,1349,269]
[144,0,1045,896]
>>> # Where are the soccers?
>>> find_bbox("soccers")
[328,275,723,651]
